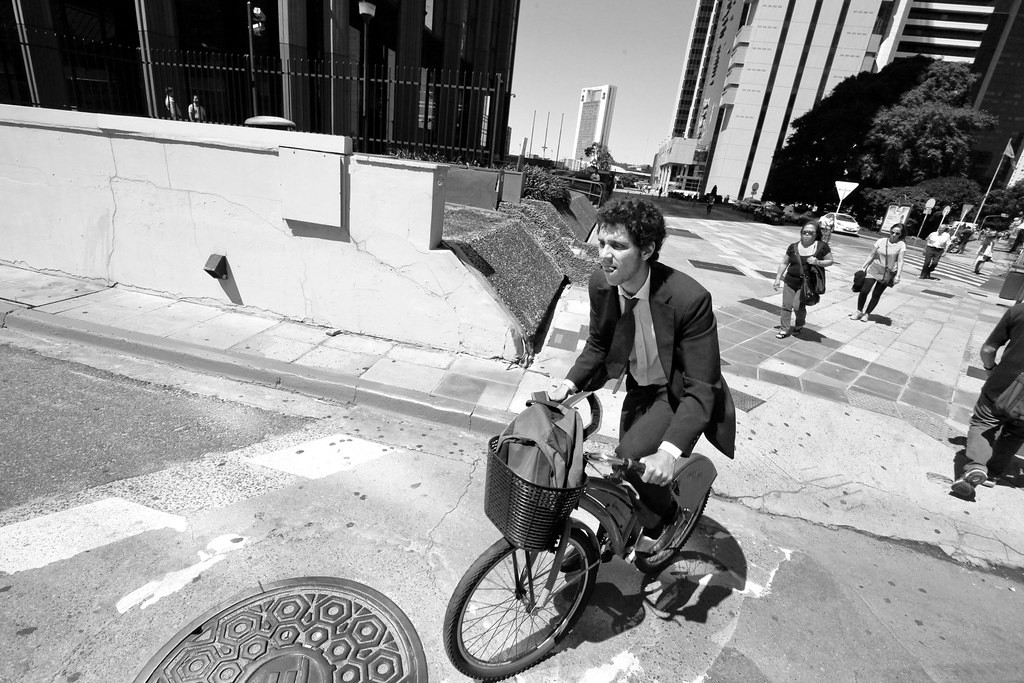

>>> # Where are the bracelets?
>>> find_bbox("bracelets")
[983,362,996,370]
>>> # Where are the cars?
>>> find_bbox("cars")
[754,203,785,225]
[819,211,860,236]
[733,199,741,210]
[782,204,812,224]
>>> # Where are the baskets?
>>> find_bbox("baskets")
[482,436,585,551]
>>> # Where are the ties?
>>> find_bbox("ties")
[605,297,640,380]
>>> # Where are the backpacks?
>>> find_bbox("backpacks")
[495,390,594,486]
[995,371,1024,426]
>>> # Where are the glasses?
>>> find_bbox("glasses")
[891,230,901,236]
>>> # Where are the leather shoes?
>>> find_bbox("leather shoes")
[632,507,686,558]
[559,546,582,573]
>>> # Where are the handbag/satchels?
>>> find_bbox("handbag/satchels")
[881,268,898,288]
[983,245,993,262]
[811,242,826,294]
[800,274,820,306]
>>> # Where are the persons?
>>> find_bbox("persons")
[973,234,995,274]
[919,224,951,280]
[850,223,906,322]
[188,95,206,122]
[707,195,715,214]
[951,302,1024,497]
[165,88,181,120]
[548,198,736,574]
[773,221,834,339]
[955,226,973,254]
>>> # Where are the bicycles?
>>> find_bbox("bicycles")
[443,393,717,682]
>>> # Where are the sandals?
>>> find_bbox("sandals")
[791,325,803,336]
[775,330,789,339]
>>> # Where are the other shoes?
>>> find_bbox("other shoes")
[950,464,989,499]
[850,310,864,320]
[983,475,998,487]
[861,314,870,322]
[918,271,931,279]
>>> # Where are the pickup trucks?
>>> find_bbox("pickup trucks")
[739,198,761,212]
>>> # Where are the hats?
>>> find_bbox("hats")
[982,232,993,237]
[966,225,971,228]
